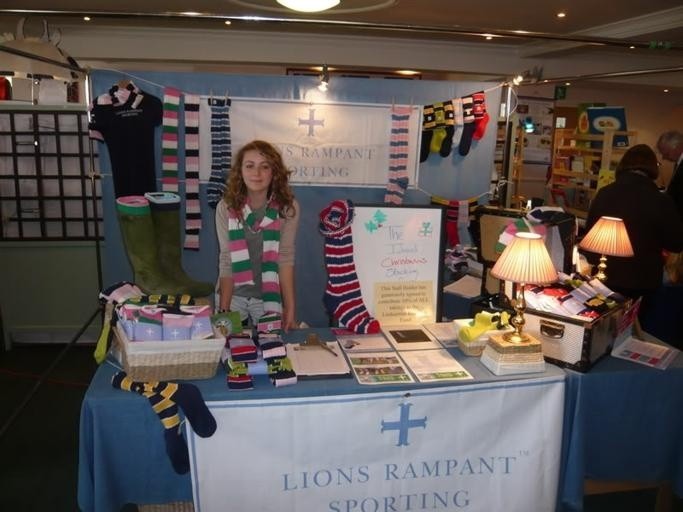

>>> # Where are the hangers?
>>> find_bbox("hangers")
[115,72,133,91]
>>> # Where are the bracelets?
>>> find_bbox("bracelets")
[218,307,231,313]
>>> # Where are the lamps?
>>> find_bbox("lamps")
[481,231,557,375]
[578,216,635,282]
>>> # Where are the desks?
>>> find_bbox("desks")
[564,329,682,512]
[75,328,565,512]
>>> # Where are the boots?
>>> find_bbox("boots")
[116,196,189,295]
[144,193,214,297]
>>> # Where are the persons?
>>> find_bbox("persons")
[212,141,299,331]
[581,142,682,305]
[654,128,682,202]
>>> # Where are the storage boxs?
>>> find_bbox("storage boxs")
[503,214,632,370]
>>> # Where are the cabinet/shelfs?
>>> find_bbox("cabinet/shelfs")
[546,127,638,218]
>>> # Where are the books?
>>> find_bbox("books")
[442,272,485,299]
[286,317,473,383]
